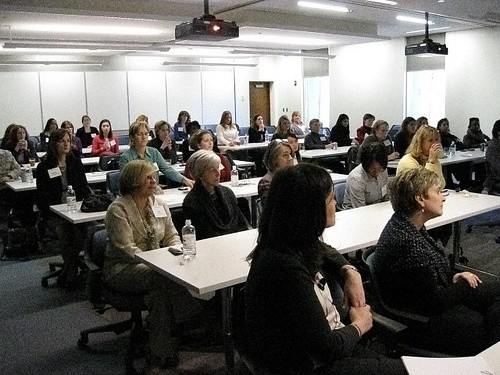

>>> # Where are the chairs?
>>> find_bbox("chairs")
[78,229,176,375]
[232,281,407,375]
[360,247,432,324]
[38,215,91,287]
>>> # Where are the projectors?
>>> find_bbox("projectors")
[175,14,239,42]
[406,39,448,55]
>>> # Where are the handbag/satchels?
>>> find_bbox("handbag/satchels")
[80,185,116,213]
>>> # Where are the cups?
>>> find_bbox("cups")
[29,158,36,166]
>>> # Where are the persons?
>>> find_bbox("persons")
[244,163,409,375]
[36,130,89,292]
[374,166,500,357]
[0,110,241,190]
[102,160,204,375]
[248,110,500,194]
[342,141,393,209]
[396,125,445,192]
[182,149,254,302]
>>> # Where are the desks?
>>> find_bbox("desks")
[0,136,500,375]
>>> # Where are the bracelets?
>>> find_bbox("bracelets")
[341,265,358,274]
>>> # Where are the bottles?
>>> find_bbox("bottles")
[244,134,248,146]
[66,185,78,215]
[449,141,456,160]
[265,130,269,144]
[230,165,238,187]
[25,165,34,185]
[181,219,196,257]
